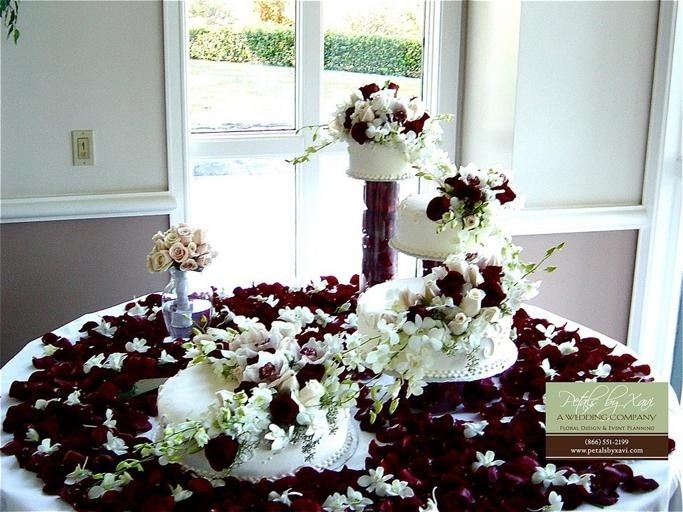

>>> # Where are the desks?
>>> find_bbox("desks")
[0,249,683,510]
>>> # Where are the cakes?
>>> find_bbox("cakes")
[93,81,566,482]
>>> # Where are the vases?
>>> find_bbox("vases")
[389,190,475,266]
[345,142,416,182]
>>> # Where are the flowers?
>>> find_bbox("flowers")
[288,79,458,176]
[146,223,216,278]
[419,144,526,233]
